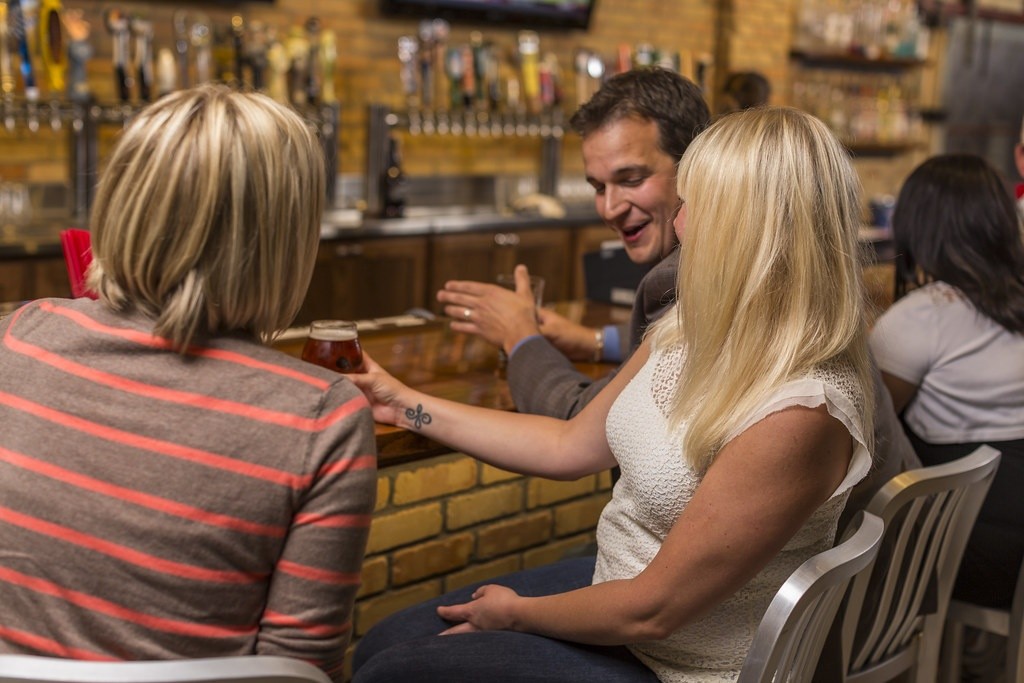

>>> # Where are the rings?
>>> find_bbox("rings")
[464,309,472,322]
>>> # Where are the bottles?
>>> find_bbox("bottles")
[378,136,408,219]
[302,319,366,377]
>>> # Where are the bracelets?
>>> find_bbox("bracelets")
[594,329,602,364]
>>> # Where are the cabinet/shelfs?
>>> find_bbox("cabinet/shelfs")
[782,1,936,158]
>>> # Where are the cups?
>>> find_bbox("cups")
[492,273,546,382]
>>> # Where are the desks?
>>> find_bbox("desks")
[265,298,636,683]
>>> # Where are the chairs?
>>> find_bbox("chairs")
[735,510,886,683]
[837,442,1002,683]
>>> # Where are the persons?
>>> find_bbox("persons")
[330,107,882,683]
[867,152,1024,611]
[440,62,950,642]
[724,73,770,110]
[1,84,378,681]
